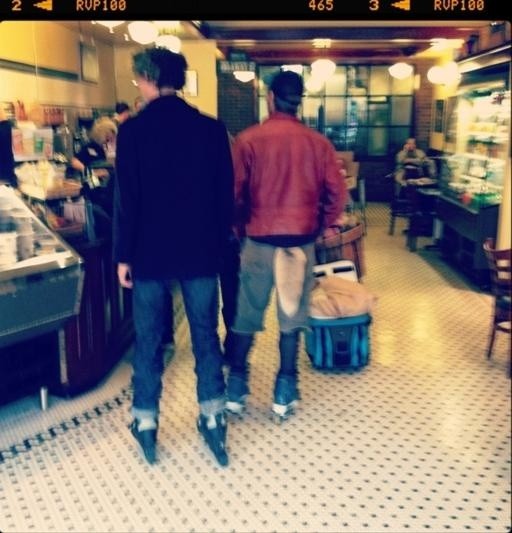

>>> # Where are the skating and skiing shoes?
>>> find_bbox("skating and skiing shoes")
[132,419,161,463]
[224,374,249,418]
[195,413,227,465]
[270,375,299,418]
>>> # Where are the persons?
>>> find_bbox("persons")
[72,116,118,164]
[108,43,238,453]
[110,100,129,129]
[133,95,144,112]
[224,70,346,418]
[396,136,427,197]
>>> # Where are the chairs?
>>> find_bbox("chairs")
[480,235,512,380]
